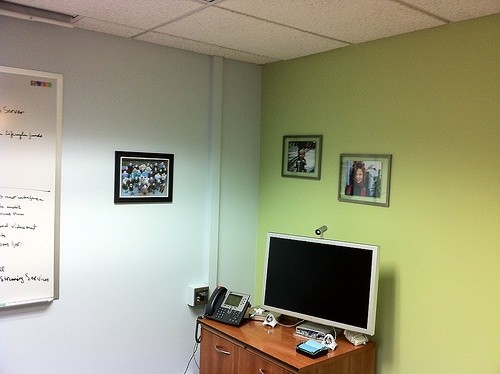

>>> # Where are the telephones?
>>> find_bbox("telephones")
[203,286,251,326]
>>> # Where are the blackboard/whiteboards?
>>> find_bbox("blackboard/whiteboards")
[0,66,64,309]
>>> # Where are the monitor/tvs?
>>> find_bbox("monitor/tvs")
[262,233,379,336]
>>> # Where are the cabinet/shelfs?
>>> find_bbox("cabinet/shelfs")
[198,314,377,374]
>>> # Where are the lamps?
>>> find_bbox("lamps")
[0,1,76,29]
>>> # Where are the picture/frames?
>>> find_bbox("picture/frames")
[338,153,392,208]
[282,135,323,180]
[114,151,174,203]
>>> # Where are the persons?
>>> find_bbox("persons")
[122,161,167,196]
[345,162,370,196]
[294,145,307,168]
